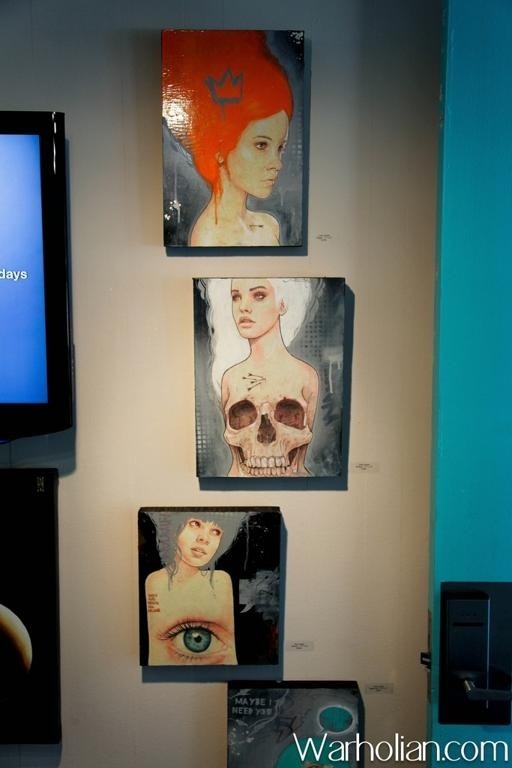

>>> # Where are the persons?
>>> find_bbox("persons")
[138,509,256,665]
[161,30,297,250]
[196,275,319,478]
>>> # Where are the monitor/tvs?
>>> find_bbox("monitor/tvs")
[0,110,74,446]
